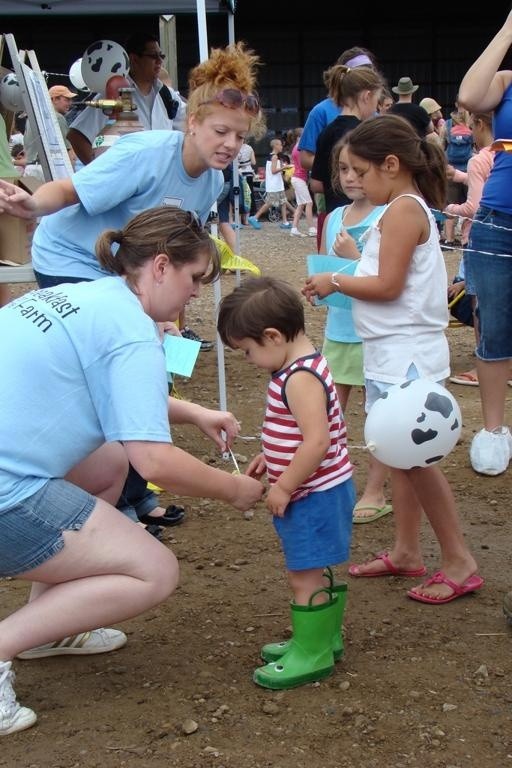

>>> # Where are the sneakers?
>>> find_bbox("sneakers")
[309,227,318,236]
[443,239,461,250]
[248,217,261,229]
[280,222,292,228]
[16,629,127,659]
[291,229,307,238]
[0,662,37,737]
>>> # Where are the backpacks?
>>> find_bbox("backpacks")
[446,119,474,165]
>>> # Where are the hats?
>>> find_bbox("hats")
[420,98,441,115]
[392,77,419,95]
[49,86,77,99]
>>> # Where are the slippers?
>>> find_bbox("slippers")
[348,553,426,577]
[408,572,484,603]
[352,500,393,523]
[450,369,479,386]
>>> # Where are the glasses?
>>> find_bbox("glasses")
[198,89,259,116]
[139,53,166,60]
[163,210,201,243]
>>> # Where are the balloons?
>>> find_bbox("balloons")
[69,56,90,93]
[81,39,131,93]
[0,73,27,113]
[364,378,463,470]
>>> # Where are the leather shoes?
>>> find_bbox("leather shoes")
[138,505,184,525]
[137,523,164,541]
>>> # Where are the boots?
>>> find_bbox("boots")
[261,579,347,663]
[253,589,338,689]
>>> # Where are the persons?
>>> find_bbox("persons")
[2,27,495,412]
[1,205,265,737]
[456,10,511,477]
[216,275,361,691]
[299,113,484,606]
